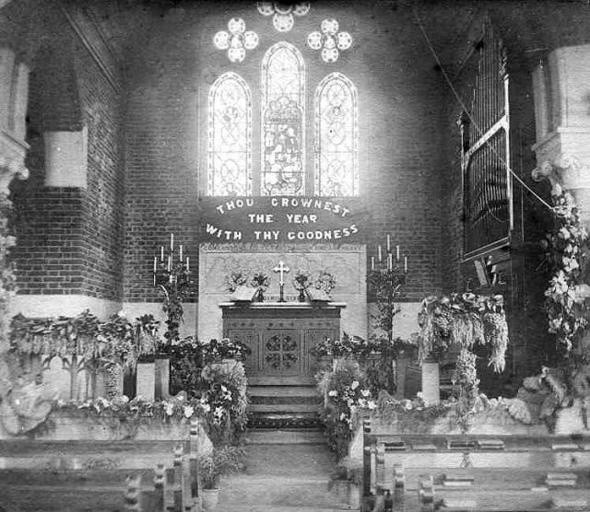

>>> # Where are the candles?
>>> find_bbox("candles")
[371,234,408,273]
[152,233,190,273]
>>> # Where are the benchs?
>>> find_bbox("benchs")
[2,399,202,512]
[351,399,590,511]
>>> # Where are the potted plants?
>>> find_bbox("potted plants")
[196,442,246,512]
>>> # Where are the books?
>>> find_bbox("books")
[306,288,332,303]
[230,286,259,301]
